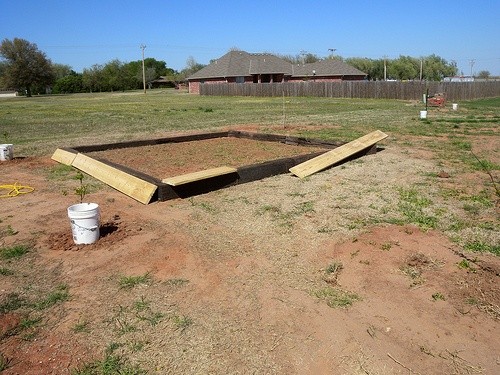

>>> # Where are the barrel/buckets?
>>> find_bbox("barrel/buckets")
[452,103,458,109]
[420,110,427,118]
[67,202,102,244]
[1,142,13,161]
